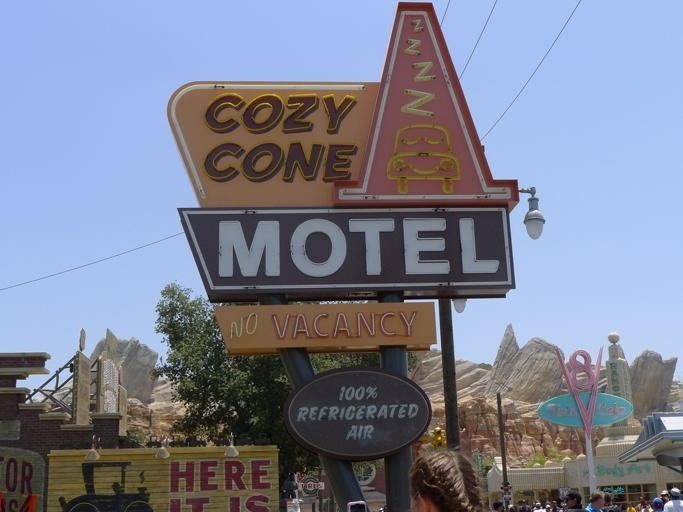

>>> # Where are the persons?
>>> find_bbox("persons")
[410,451,479,512]
[493,486,683,512]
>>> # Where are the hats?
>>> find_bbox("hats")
[671,488,680,496]
[661,491,669,495]
[561,490,582,503]
[653,498,664,508]
